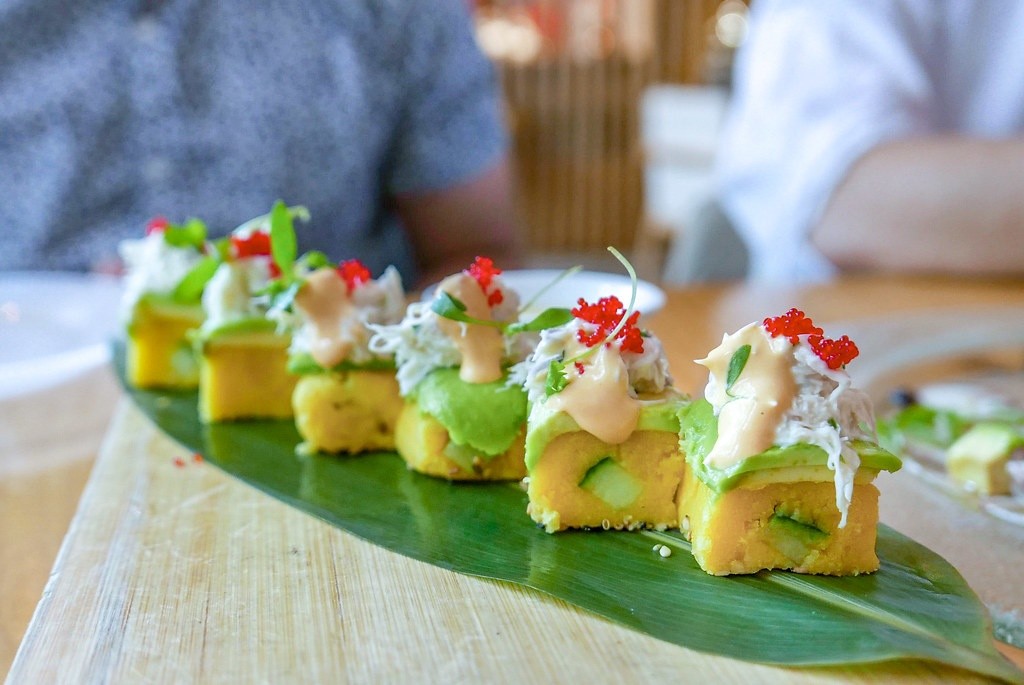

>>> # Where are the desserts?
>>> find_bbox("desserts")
[116,197,904,577]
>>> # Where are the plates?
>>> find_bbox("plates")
[424,268,663,340]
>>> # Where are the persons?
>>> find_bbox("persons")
[716,0,1024,284]
[0,1,524,293]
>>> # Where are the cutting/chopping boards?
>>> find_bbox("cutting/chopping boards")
[4,377,1024,685]
